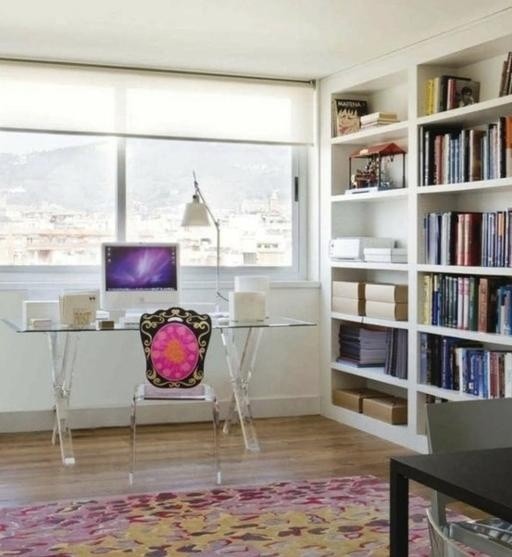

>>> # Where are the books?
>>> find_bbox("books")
[332,236,407,264]
[418,47,512,402]
[335,98,400,136]
[336,324,407,380]
[331,281,408,320]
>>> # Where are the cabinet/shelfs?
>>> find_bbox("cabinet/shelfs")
[321,8,512,454]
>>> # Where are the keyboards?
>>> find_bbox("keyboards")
[124,322,140,328]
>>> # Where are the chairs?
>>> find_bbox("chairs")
[127,307,223,487]
[422,397,512,556]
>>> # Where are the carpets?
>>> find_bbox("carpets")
[0,471,488,557]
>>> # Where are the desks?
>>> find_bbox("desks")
[1,307,317,462]
[388,449,512,556]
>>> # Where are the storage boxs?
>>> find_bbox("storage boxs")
[333,387,407,426]
[330,279,407,322]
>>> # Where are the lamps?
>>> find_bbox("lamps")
[178,168,227,302]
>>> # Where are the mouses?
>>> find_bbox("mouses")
[218,318,228,325]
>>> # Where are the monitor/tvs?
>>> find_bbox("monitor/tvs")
[101,242,180,319]
